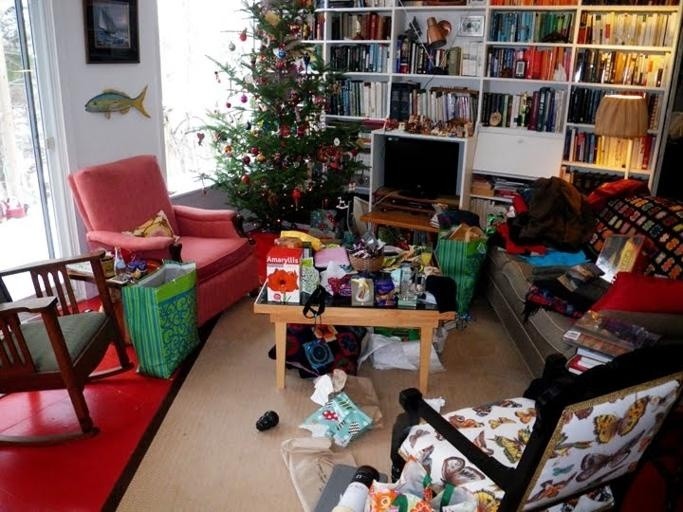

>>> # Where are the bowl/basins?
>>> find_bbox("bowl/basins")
[348,249,384,272]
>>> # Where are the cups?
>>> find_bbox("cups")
[420,241,433,267]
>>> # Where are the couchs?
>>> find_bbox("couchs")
[481,179,682,380]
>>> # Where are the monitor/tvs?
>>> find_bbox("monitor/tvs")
[384,135,459,200]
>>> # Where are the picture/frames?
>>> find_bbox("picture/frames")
[82,0,140,64]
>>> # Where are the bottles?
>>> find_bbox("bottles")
[112,246,126,281]
[256,411,279,431]
[128,259,147,280]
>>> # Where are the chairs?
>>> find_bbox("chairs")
[389,333,682,510]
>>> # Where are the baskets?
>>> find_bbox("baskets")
[348,253,384,273]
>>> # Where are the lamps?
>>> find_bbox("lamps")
[593,91,651,179]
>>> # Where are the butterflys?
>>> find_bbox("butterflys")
[409,386,678,512]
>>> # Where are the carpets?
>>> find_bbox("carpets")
[97,287,532,512]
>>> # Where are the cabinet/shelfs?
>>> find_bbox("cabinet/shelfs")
[257,0,683,256]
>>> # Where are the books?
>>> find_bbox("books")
[471,177,527,231]
[560,309,659,376]
[568,84,664,130]
[327,77,389,118]
[257,1,324,187]
[390,83,480,124]
[573,48,671,88]
[486,45,572,80]
[397,39,484,77]
[560,128,658,196]
[482,87,568,134]
[577,1,679,48]
[325,121,377,185]
[330,44,392,73]
[406,228,437,246]
[328,1,392,40]
[489,0,578,43]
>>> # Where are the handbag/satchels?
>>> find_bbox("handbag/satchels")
[435,228,487,316]
[120,261,202,381]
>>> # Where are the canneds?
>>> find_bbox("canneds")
[99,256,115,279]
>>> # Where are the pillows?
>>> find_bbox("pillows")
[589,272,683,313]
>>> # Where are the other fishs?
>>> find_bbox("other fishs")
[84,84,152,119]
